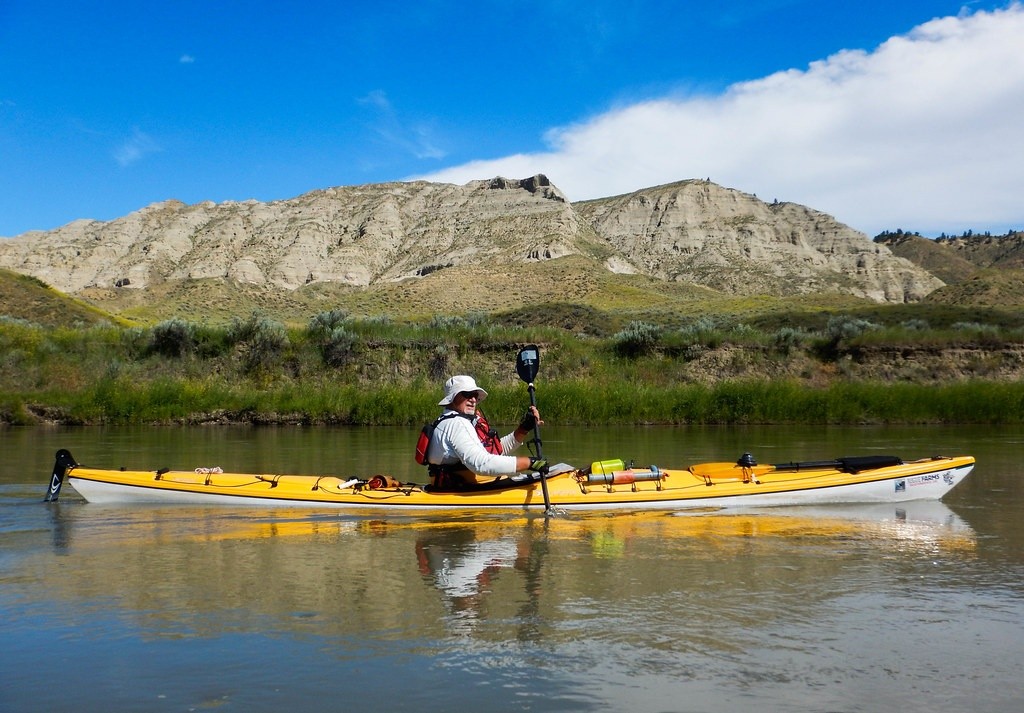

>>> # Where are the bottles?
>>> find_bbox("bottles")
[591,459,625,475]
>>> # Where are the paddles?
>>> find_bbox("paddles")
[689,454,904,480]
[515,343,553,512]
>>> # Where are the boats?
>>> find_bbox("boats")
[55,449,976,515]
[57,503,977,553]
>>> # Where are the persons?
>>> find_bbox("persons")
[413,531,529,636]
[415,375,550,493]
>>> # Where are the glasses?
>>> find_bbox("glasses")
[459,391,478,399]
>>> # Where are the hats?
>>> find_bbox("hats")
[438,376,488,406]
